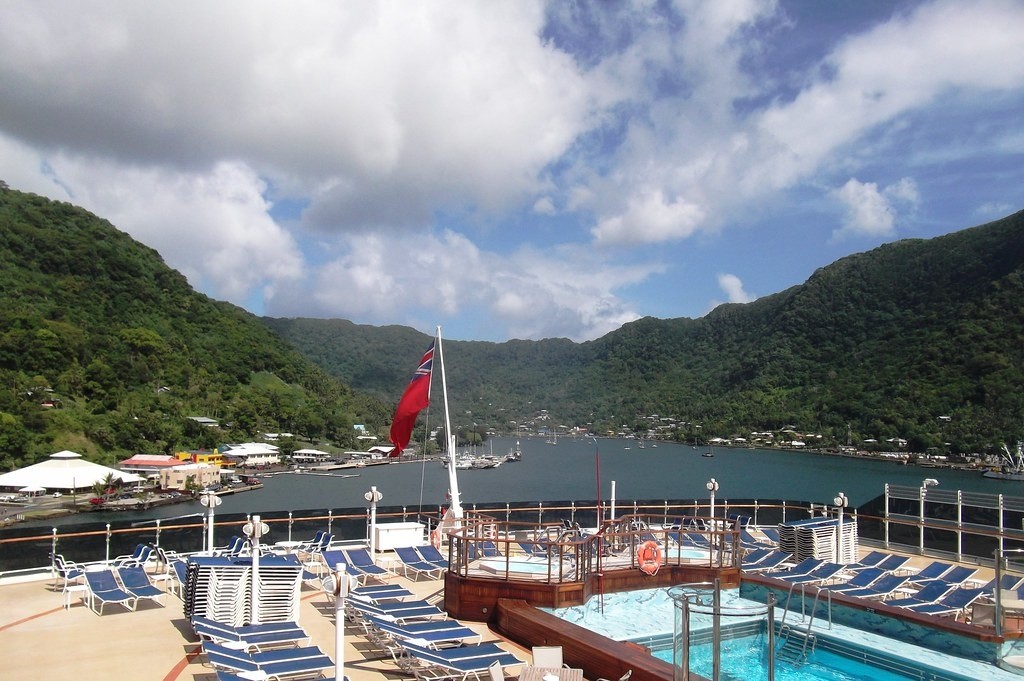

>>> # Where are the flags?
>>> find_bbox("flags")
[389,338,436,457]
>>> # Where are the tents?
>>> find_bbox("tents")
[19,486,46,498]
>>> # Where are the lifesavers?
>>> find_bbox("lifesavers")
[635,541,663,572]
[430,531,441,550]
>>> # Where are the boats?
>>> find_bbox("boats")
[437,425,523,470]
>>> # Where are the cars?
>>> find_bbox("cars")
[160,478,260,498]
[88,484,155,504]
[52,491,63,498]
[0,493,30,503]
